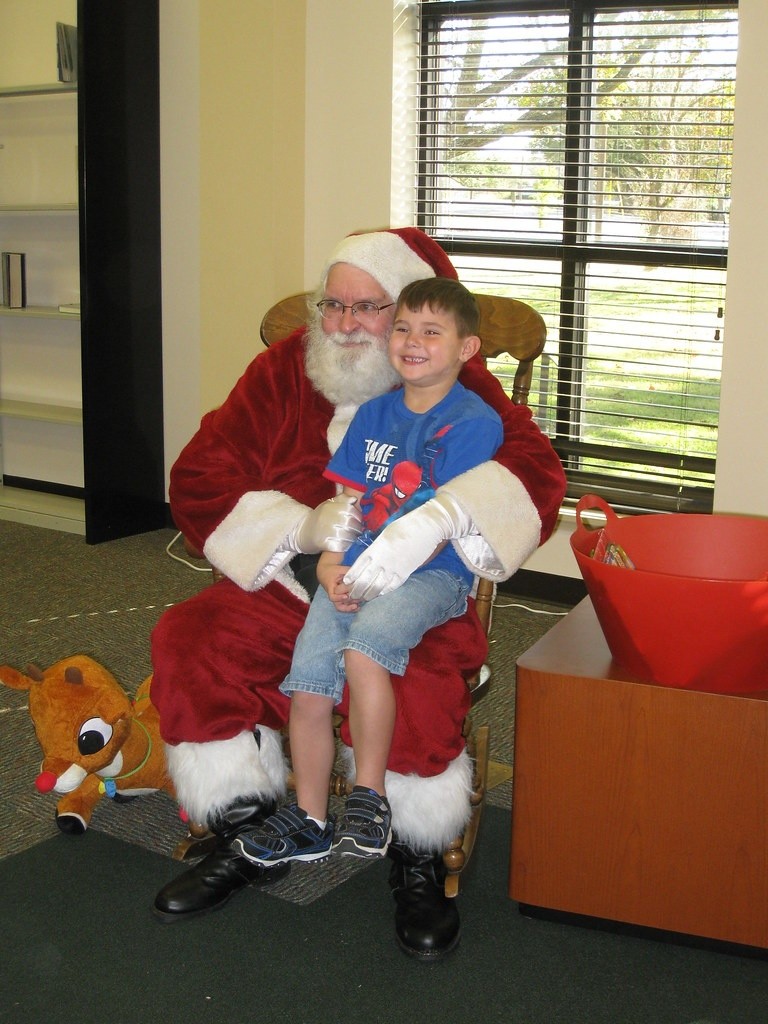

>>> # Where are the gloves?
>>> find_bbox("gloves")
[281,494,363,554]
[342,492,479,603]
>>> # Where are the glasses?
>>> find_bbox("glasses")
[317,297,396,318]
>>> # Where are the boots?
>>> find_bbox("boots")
[151,796,290,925]
[387,830,464,960]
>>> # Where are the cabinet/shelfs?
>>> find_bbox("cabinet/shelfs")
[508,592,768,956]
[1,81,85,499]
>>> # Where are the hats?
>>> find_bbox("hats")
[326,226,461,309]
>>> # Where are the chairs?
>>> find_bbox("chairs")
[171,291,546,900]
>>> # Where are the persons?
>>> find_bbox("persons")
[230,280,510,869]
[144,222,572,963]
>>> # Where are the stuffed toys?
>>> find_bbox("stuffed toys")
[1,654,178,836]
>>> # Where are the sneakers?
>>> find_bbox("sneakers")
[230,786,394,867]
